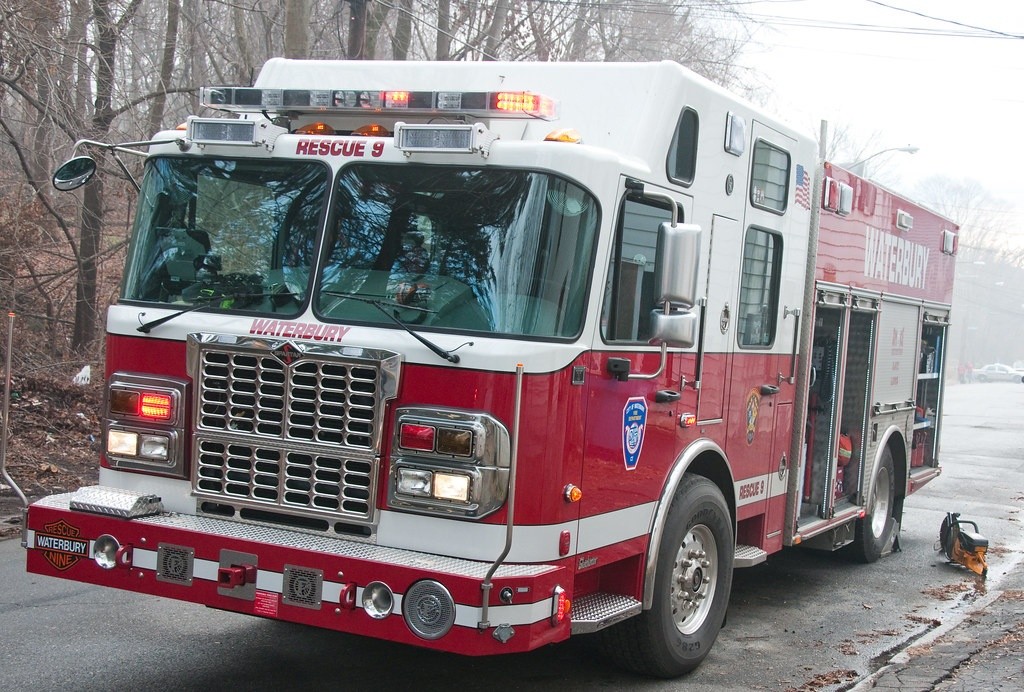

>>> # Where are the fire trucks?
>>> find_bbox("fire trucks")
[17,56,963,676]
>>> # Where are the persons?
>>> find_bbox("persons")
[957,360,974,385]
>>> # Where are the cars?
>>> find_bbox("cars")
[974,364,1024,384]
